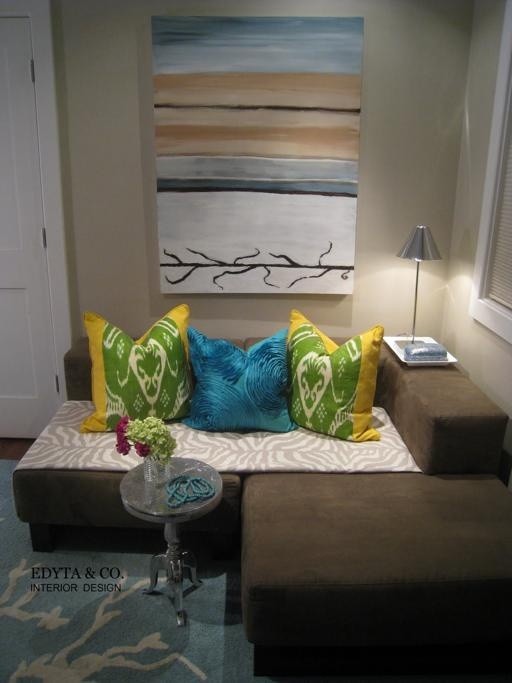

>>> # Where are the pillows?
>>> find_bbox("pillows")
[178,327,300,434]
[288,308,385,443]
[80,303,194,435]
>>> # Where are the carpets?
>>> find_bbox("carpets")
[1,457,254,681]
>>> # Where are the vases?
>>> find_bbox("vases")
[137,451,170,483]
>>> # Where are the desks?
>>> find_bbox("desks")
[119,456,224,627]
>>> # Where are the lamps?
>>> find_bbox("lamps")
[394,223,444,351]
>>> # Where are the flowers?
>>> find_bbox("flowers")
[113,411,177,464]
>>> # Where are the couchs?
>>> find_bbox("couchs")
[241,472,512,677]
[11,335,511,553]
